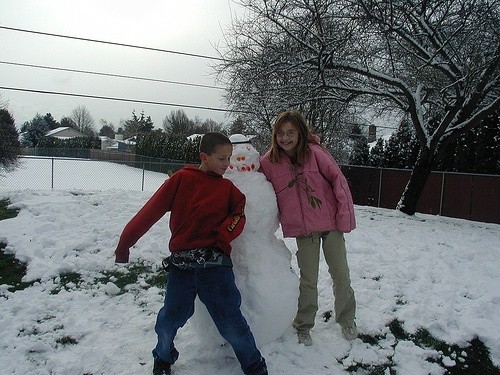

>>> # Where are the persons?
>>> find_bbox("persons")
[257,110,357,349]
[114,132,269,375]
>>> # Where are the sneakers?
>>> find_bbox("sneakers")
[297,329,312,346]
[341,322,359,340]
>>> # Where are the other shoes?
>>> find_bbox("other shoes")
[248,363,268,375]
[153,358,171,375]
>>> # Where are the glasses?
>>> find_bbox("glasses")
[275,129,298,137]
